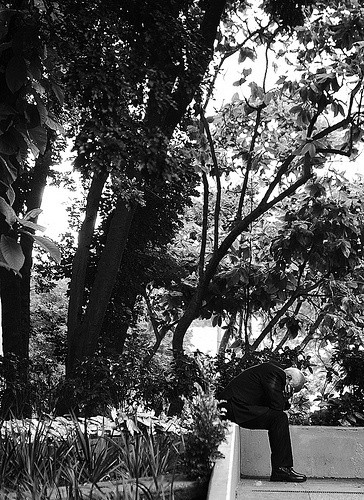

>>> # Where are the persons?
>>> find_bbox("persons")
[216,361,308,483]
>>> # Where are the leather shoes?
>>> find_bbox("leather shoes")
[270,467,307,482]
